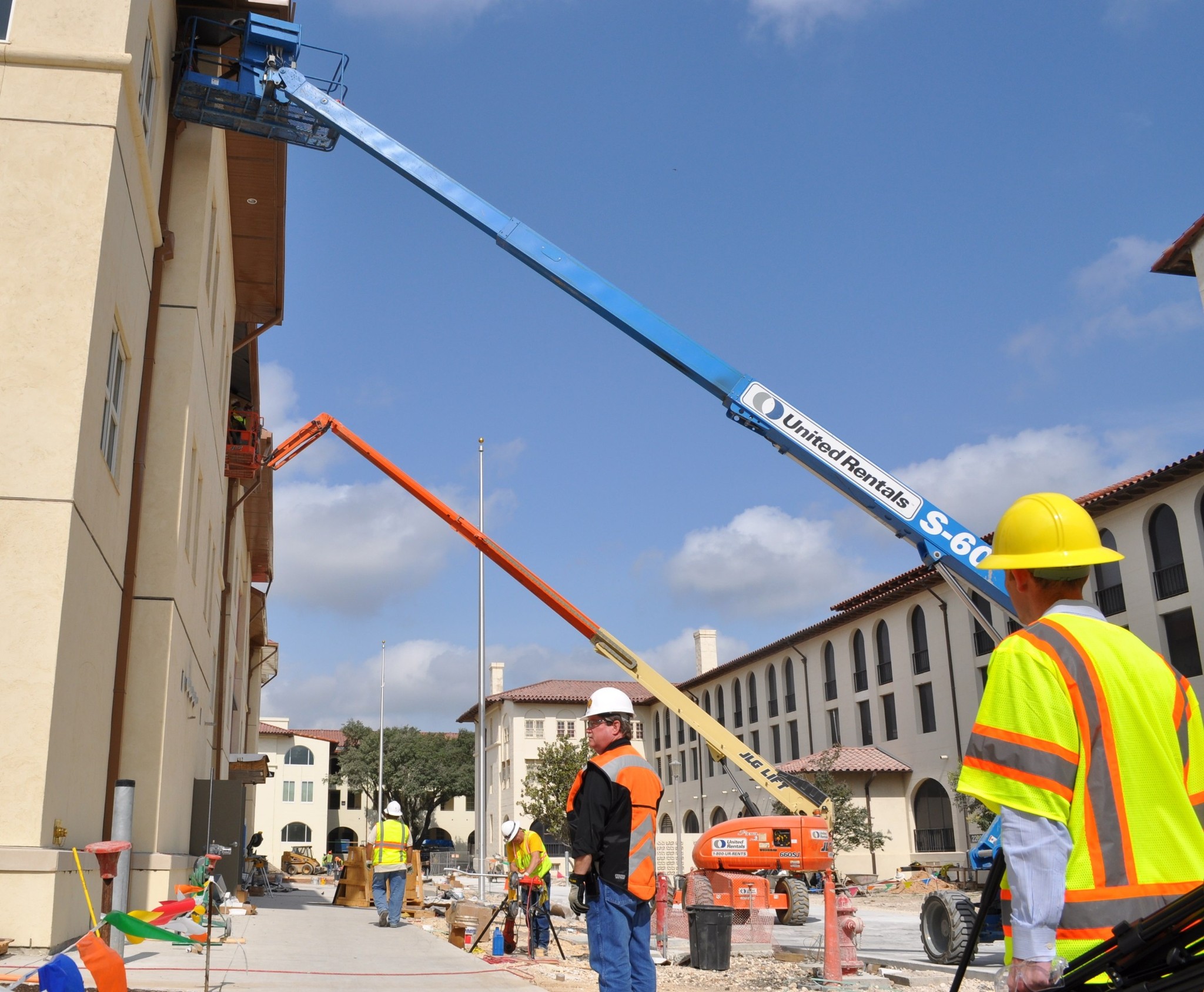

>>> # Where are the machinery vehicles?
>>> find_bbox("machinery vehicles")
[280,845,325,875]
[222,393,836,928]
[165,10,1019,969]
[414,839,455,877]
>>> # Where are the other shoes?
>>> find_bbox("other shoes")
[522,946,528,951]
[329,874,333,876]
[543,949,548,955]
[387,923,397,927]
[379,910,389,926]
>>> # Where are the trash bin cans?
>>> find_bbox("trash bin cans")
[686,905,734,971]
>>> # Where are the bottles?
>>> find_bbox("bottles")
[493,927,504,955]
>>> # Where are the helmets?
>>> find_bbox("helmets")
[329,851,331,853]
[384,801,403,816]
[579,687,637,721]
[501,821,520,842]
[334,857,339,861]
[976,492,1124,569]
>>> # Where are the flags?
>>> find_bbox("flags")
[38,881,209,992]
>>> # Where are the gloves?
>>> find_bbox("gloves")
[366,860,372,871]
[518,872,529,879]
[406,863,413,875]
[569,873,589,916]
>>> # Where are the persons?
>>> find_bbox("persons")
[502,821,552,955]
[231,399,246,444]
[324,851,334,876]
[322,853,327,867]
[230,19,246,38]
[367,800,414,927]
[335,857,343,867]
[956,493,1203,991]
[567,687,665,992]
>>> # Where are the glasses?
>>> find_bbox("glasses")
[587,720,609,729]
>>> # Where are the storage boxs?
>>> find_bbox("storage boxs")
[236,890,247,903]
[449,902,493,943]
[249,886,264,896]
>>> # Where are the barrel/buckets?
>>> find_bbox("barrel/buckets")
[455,916,479,949]
[319,878,326,885]
[275,873,282,883]
[311,877,318,884]
[685,905,734,970]
